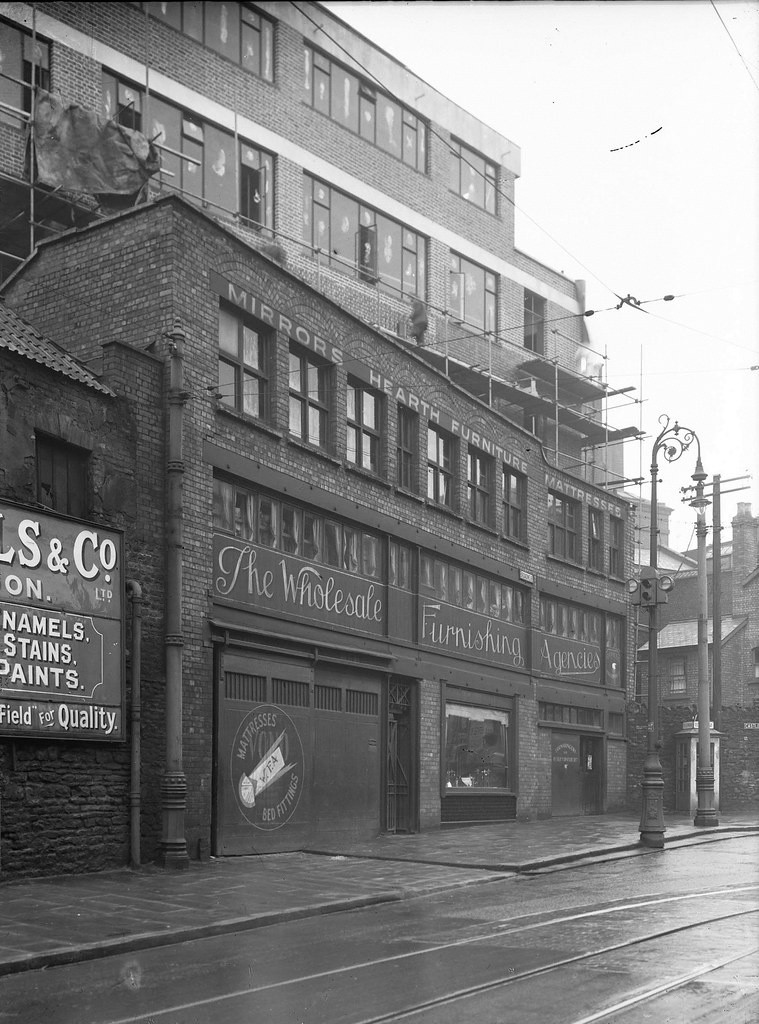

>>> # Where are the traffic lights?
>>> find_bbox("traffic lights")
[639,567,657,607]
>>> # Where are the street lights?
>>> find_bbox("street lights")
[640,414,710,848]
